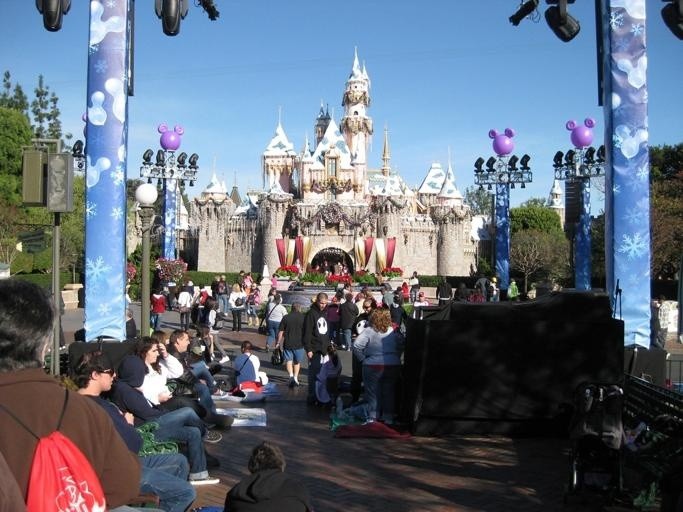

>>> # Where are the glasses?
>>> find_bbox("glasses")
[362,306,371,309]
[98,366,114,377]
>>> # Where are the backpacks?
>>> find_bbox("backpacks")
[232,292,242,307]
[211,309,223,329]
[1,387,108,512]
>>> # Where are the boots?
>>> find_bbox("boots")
[203,410,234,427]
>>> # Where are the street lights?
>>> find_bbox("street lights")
[133,181,159,338]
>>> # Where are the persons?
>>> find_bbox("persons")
[0,278,145,511]
[150,263,261,333]
[266,260,429,423]
[71,350,197,512]
[73,332,267,485]
[220,439,312,511]
[574,342,625,485]
[436,275,563,302]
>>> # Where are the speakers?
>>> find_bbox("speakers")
[624,350,666,389]
[46,152,73,213]
[22,151,47,207]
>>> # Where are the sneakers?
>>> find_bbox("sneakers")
[287,377,295,387]
[210,389,229,399]
[218,355,229,363]
[291,376,298,386]
[188,475,220,485]
[202,430,222,443]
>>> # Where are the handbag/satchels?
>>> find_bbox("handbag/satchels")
[271,346,283,365]
[257,326,267,335]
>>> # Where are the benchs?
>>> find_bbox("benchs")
[622,373,683,491]
